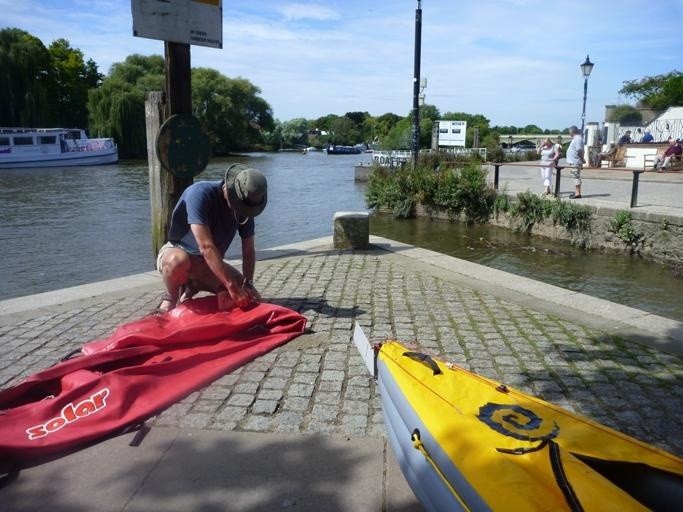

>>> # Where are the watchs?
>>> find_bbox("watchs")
[242,278,255,288]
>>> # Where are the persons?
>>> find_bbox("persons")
[631,128,642,144]
[144,163,263,316]
[616,130,631,146]
[565,125,586,200]
[533,138,558,196]
[653,138,682,173]
[640,128,654,142]
[554,138,561,167]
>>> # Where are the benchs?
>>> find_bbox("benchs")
[594,146,626,167]
[644,152,683,172]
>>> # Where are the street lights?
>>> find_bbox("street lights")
[579,54,594,134]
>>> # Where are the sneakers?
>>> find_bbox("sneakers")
[569,193,581,199]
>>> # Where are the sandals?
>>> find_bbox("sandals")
[151,278,199,316]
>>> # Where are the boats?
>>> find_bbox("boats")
[0,126,119,170]
[349,321,683,512]
[326,144,360,154]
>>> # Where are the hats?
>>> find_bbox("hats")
[225,161,268,220]
